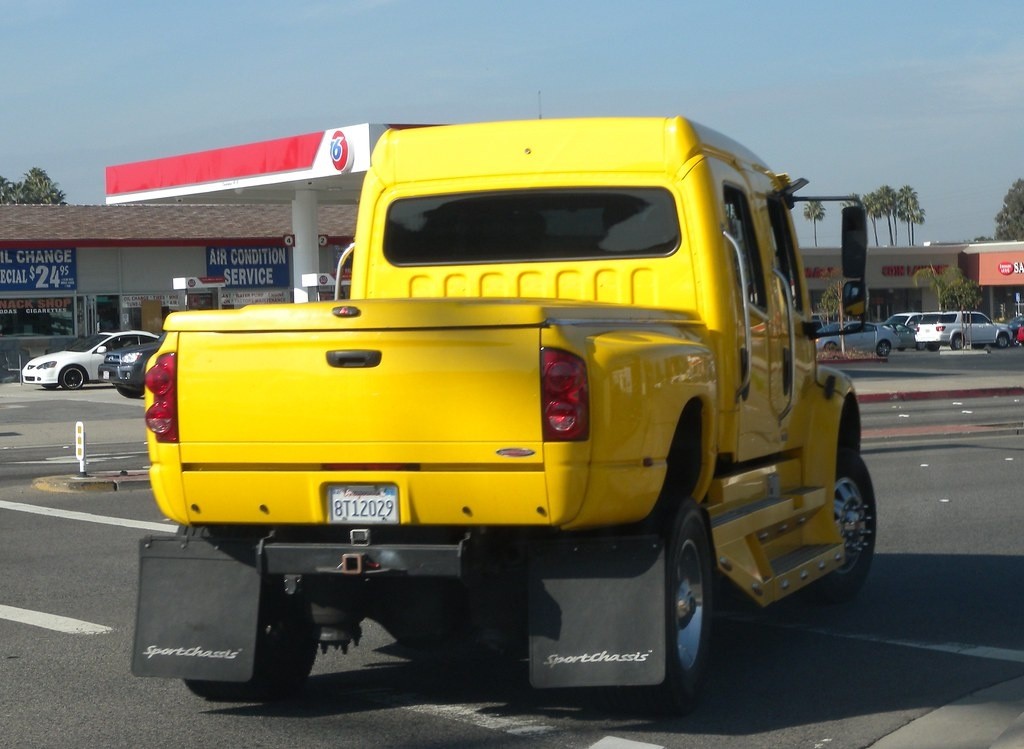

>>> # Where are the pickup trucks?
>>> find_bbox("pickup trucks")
[131,113,879,715]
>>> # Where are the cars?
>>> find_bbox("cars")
[883,312,929,352]
[814,320,901,358]
[22,329,160,391]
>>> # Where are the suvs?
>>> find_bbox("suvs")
[98,331,167,398]
[914,310,1014,351]
